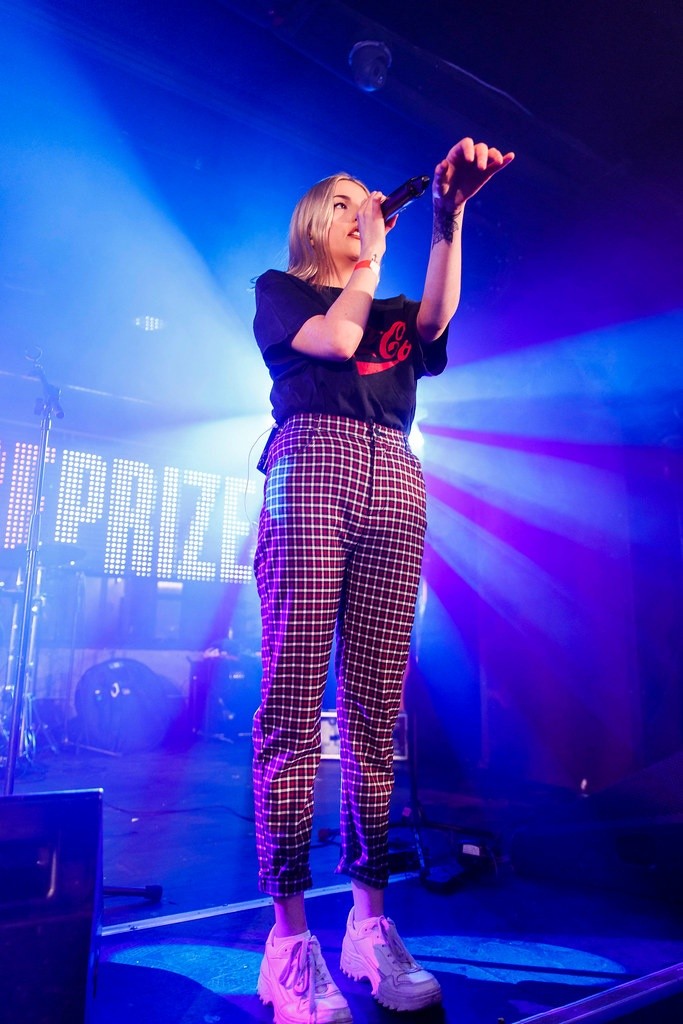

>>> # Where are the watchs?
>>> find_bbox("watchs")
[354,259,380,285]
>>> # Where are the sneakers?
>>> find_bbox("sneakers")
[340,905,440,1011]
[257,923,352,1023]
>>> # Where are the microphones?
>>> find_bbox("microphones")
[378,175,431,223]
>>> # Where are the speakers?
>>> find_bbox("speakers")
[0,788,106,1024]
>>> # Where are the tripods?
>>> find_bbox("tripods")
[319,624,497,879]
[36,565,123,759]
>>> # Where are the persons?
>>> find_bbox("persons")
[249,137,517,1024]
